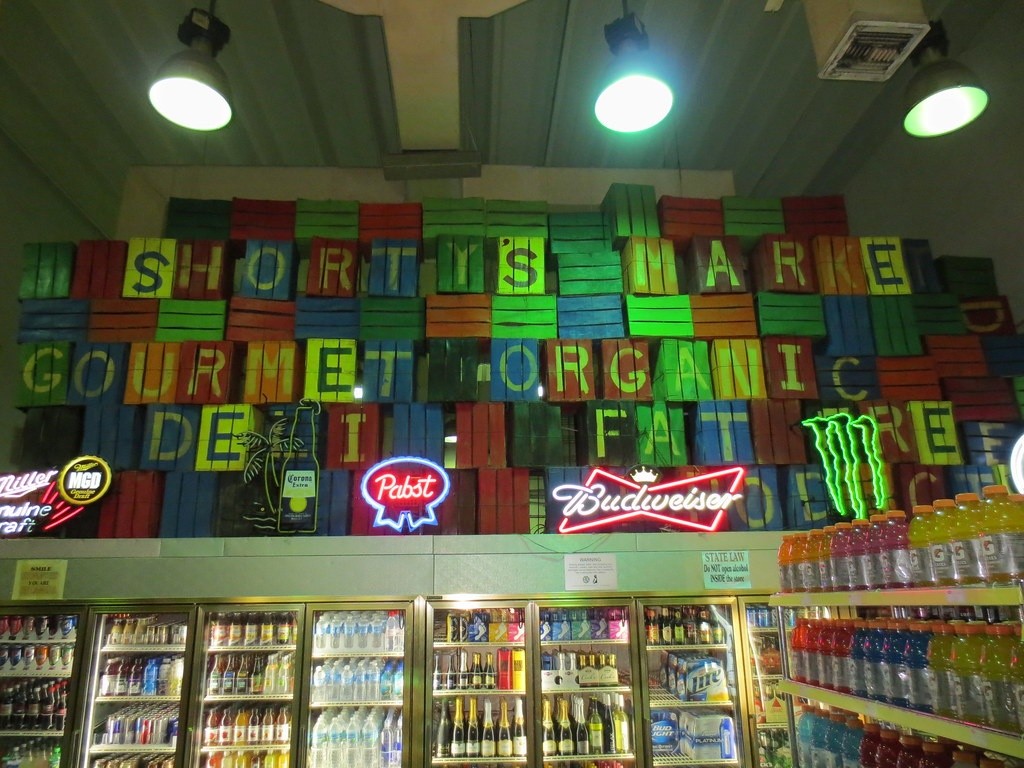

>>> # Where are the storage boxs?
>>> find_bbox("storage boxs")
[649,650,734,760]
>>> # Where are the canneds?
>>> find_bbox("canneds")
[746,603,829,627]
[0,615,76,668]
[92,753,174,768]
[105,701,177,745]
[147,620,188,644]
[445,609,508,644]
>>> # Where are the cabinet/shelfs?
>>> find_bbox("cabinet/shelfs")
[766,587,1024,768]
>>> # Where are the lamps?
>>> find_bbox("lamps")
[593,0,675,134]
[901,16,991,138]
[147,0,233,133]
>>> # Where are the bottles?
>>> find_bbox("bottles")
[644,605,724,645]
[0,678,69,768]
[203,611,297,768]
[776,485,1024,768]
[98,617,187,697]
[540,649,630,768]
[750,636,792,768]
[719,718,733,759]
[309,611,404,768]
[433,646,528,768]
